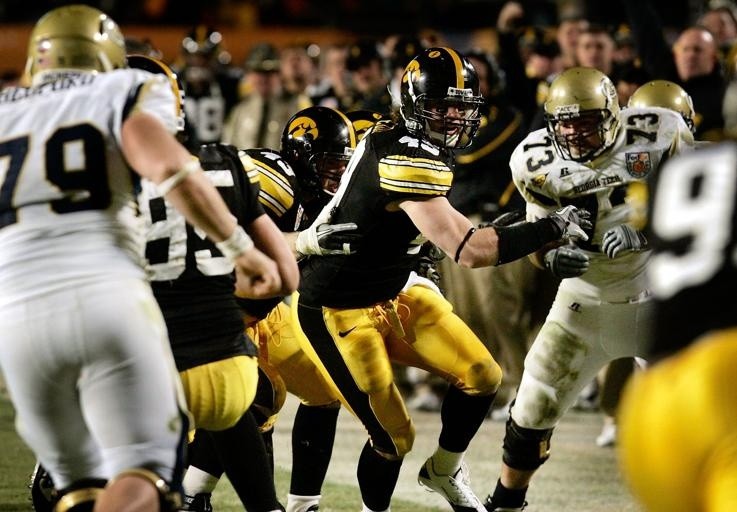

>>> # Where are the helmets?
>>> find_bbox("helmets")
[400,44,483,125]
[543,65,622,148]
[23,2,130,85]
[280,105,360,186]
[123,53,188,124]
[343,109,385,148]
[623,77,695,133]
[182,25,222,65]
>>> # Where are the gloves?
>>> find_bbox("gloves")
[477,207,529,228]
[600,223,652,262]
[544,202,595,244]
[293,194,368,259]
[535,236,591,281]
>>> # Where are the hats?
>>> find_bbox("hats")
[245,41,283,74]
[344,39,382,70]
[391,35,426,68]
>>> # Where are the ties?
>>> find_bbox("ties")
[254,102,271,148]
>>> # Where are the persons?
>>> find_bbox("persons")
[1,1,736,511]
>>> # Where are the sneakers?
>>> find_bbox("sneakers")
[482,494,529,512]
[595,422,617,448]
[416,454,489,512]
